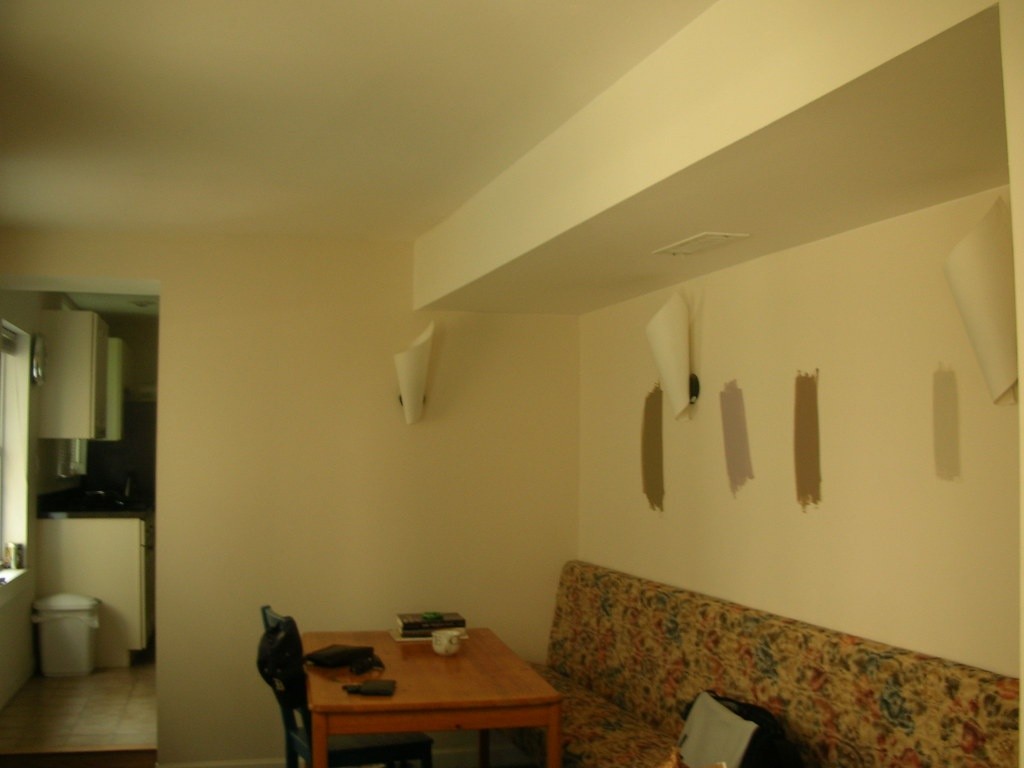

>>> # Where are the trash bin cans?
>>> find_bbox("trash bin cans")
[32,592,102,678]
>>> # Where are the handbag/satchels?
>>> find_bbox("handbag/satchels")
[681,688,807,768]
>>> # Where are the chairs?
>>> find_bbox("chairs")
[261,605,435,768]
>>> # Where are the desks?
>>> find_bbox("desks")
[303,627,562,768]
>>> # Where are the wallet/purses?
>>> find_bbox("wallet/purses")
[362,679,395,696]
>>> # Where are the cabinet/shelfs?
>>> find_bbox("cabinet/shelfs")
[36,508,155,670]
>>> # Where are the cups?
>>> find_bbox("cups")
[431,630,459,655]
[6,543,22,569]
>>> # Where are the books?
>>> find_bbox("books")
[389,611,469,642]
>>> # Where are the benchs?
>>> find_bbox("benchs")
[487,560,1024,767]
